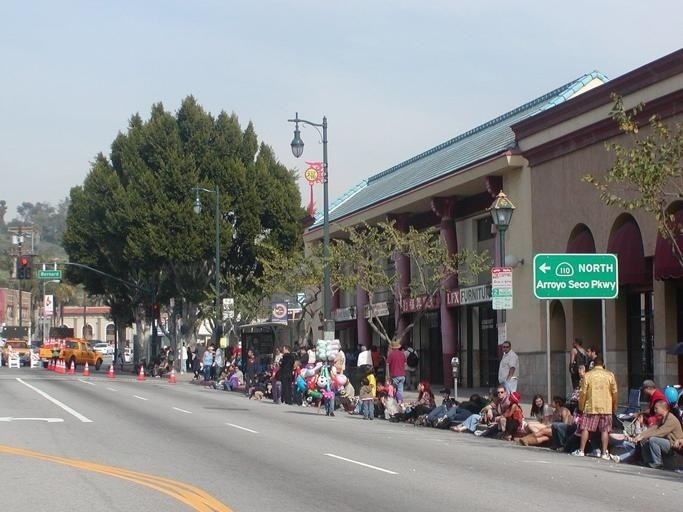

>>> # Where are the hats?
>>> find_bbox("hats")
[638,380,655,390]
[388,342,400,349]
[508,392,520,405]
[440,387,451,394]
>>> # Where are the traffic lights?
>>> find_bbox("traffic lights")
[16,255,32,279]
[150,303,159,319]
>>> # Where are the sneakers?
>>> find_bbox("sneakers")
[571,450,585,457]
[601,453,610,460]
[589,450,601,458]
[609,454,620,464]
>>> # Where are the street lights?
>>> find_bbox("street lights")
[15,226,36,340]
[486,189,516,341]
[189,182,222,350]
[287,110,331,319]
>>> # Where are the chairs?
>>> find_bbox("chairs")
[613,387,641,438]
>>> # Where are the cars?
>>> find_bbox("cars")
[92,342,113,354]
[111,347,133,364]
[87,339,101,346]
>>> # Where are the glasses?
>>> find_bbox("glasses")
[502,346,510,348]
[497,392,503,394]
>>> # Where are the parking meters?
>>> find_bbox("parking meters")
[448,356,460,400]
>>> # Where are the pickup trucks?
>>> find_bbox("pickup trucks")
[38,338,103,371]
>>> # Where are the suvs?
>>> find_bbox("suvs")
[0,340,31,366]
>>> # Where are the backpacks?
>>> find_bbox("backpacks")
[406,350,418,368]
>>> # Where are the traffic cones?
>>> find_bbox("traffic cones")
[136,365,145,381]
[168,367,176,384]
[42,335,68,349]
[66,359,76,374]
[46,358,66,374]
[105,363,115,379]
[81,361,91,376]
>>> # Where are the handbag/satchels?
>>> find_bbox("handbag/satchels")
[572,347,586,372]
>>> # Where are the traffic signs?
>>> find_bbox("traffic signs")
[533,253,619,299]
[37,271,62,279]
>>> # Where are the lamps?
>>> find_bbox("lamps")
[502,254,525,269]
[316,311,327,327]
[347,304,359,320]
[385,293,396,316]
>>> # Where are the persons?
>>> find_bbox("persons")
[203,343,259,397]
[341,340,419,420]
[159,342,201,380]
[50,342,60,366]
[4,342,12,366]
[472,341,523,442]
[254,344,316,405]
[324,389,335,417]
[388,379,499,432]
[519,339,682,470]
